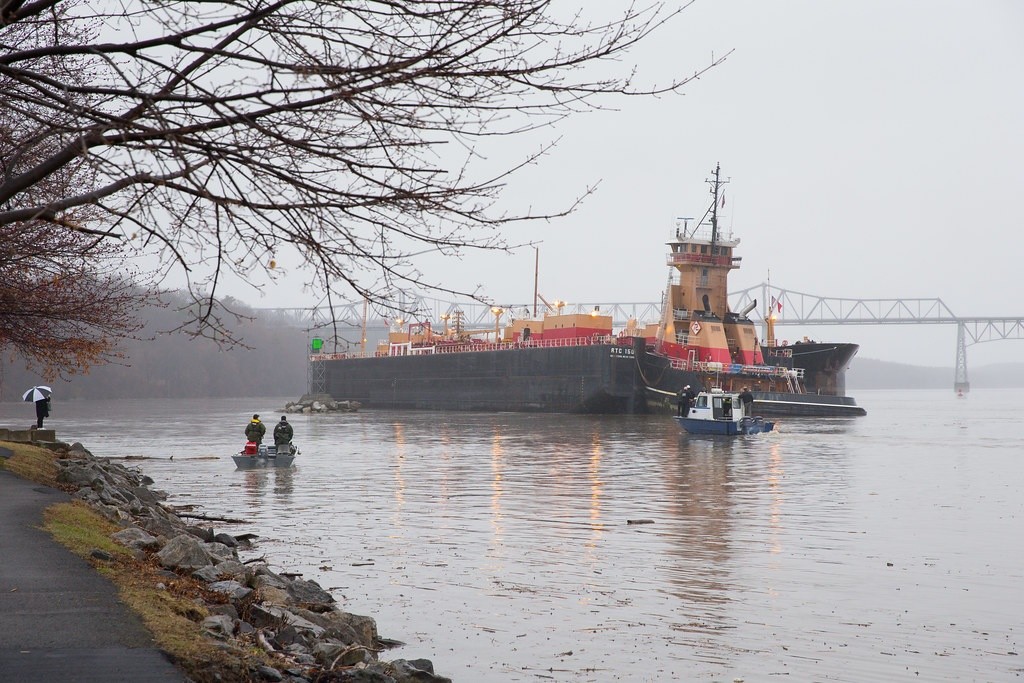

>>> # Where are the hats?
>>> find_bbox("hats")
[253,414,259,419]
[702,387,706,391]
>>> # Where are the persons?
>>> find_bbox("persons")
[274,416,293,444]
[723,398,731,416]
[678,385,695,417]
[36,396,51,428]
[698,387,707,406]
[245,414,266,444]
[738,387,754,416]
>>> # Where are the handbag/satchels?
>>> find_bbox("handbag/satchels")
[46,398,51,410]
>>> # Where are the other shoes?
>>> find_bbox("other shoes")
[37,428,46,430]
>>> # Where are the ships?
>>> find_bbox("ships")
[304,164,869,424]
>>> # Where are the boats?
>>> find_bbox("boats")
[230,444,297,469]
[671,350,774,437]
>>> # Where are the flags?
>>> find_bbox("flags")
[778,303,783,313]
[772,297,776,308]
[723,196,725,207]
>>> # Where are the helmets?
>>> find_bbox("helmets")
[684,387,688,390]
[687,385,690,388]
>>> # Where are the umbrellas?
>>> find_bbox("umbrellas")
[22,385,52,402]
